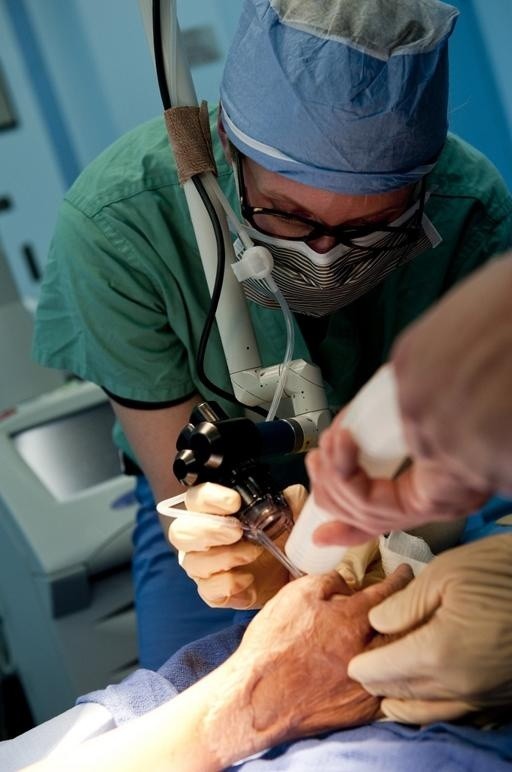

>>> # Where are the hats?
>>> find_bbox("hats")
[218,0,459,195]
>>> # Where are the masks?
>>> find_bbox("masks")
[231,167,441,317]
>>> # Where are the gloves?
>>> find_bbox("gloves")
[347,532,512,724]
[334,538,379,588]
[168,482,307,609]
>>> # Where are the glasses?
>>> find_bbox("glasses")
[232,147,426,249]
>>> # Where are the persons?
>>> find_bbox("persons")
[0,561,430,771]
[305,255,512,548]
[35,0,512,727]
